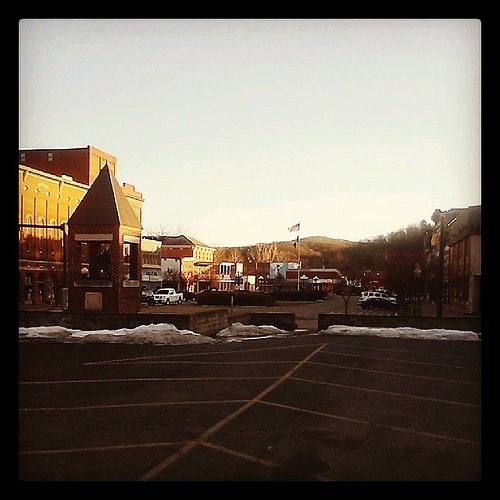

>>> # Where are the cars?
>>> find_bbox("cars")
[358,297,398,311]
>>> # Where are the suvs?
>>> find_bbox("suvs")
[361,292,397,305]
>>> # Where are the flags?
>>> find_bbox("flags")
[288,224,298,232]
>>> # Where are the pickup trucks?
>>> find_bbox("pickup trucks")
[154,288,184,305]
